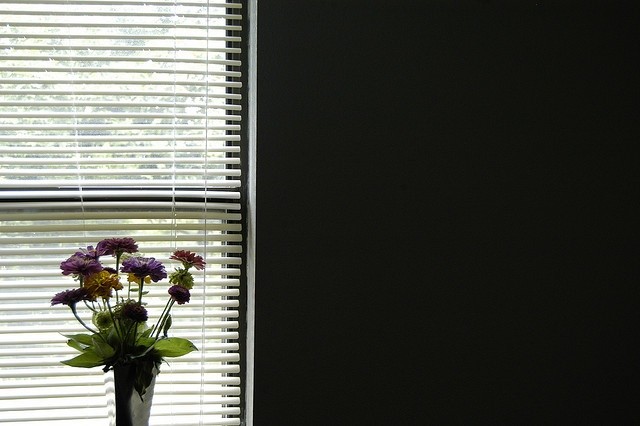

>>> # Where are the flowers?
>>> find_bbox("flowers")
[50,237,207,372]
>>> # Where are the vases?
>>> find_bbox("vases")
[104,357,161,426]
